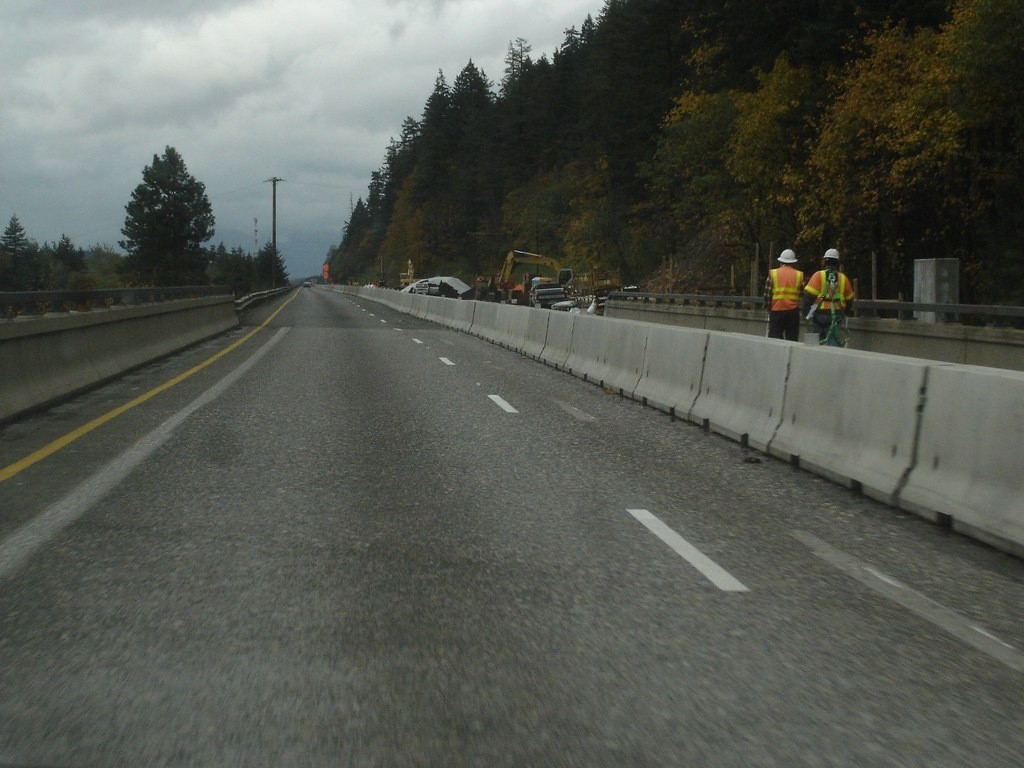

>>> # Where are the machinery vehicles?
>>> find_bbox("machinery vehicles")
[476,249,565,304]
[398,263,425,289]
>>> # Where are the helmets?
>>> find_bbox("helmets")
[777,249,798,264]
[824,249,839,259]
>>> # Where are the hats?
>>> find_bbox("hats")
[477,276,485,282]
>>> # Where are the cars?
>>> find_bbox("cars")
[304,281,310,287]
[399,276,473,298]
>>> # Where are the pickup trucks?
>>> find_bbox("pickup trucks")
[529,282,566,308]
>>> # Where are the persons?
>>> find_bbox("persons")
[762,249,807,342]
[802,249,853,347]
[475,276,491,301]
